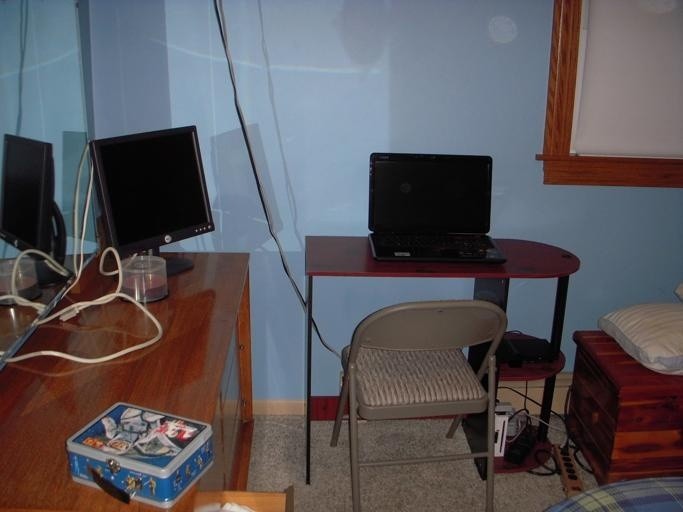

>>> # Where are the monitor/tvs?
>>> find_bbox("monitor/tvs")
[1,133,56,260]
[88,125,215,276]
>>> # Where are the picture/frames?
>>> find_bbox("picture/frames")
[533,1,683,190]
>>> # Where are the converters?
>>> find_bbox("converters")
[508,431,535,462]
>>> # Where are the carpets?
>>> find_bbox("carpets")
[248,413,599,511]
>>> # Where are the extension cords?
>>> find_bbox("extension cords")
[554,443,585,498]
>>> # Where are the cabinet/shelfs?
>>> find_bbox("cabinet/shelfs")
[1,249,253,512]
[564,330,682,482]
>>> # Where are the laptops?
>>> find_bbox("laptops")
[367,153,506,266]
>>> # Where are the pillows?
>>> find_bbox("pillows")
[598,301,682,374]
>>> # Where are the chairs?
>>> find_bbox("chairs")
[330,298,507,512]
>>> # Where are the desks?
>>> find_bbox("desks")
[305,235,581,486]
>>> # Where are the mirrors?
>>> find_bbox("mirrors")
[0,1,103,375]
